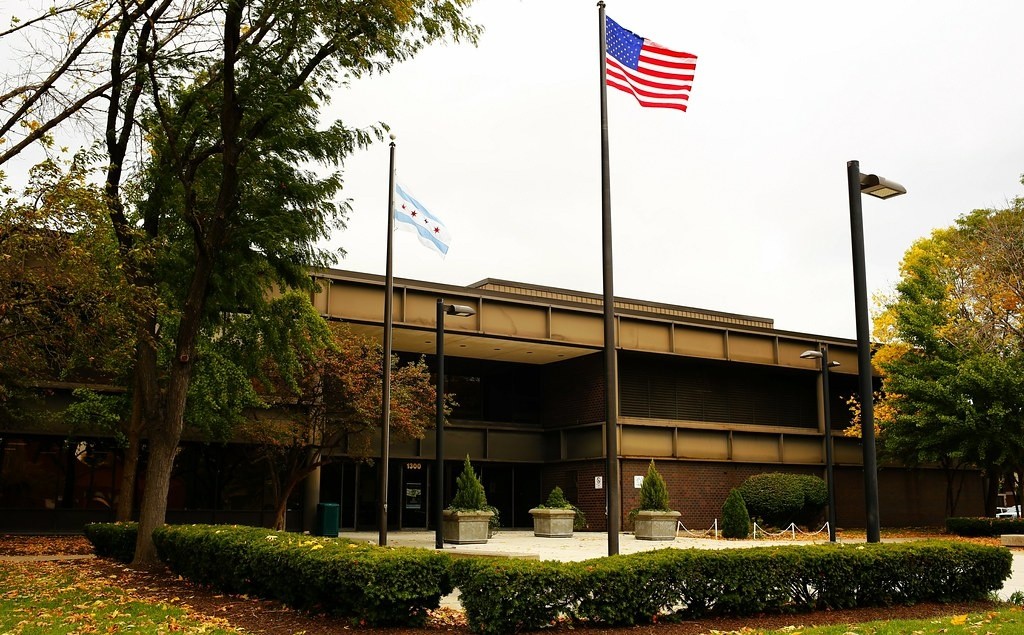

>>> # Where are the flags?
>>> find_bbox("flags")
[605,14,698,112]
[392,170,451,259]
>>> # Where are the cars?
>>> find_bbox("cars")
[995,505,1022,519]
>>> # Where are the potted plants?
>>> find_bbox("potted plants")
[527,485,587,539]
[442,450,500,543]
[627,457,683,540]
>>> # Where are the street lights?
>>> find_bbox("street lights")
[846,159,907,543]
[799,340,841,541]
[436,297,478,549]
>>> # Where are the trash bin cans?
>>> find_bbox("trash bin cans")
[317,503,339,536]
